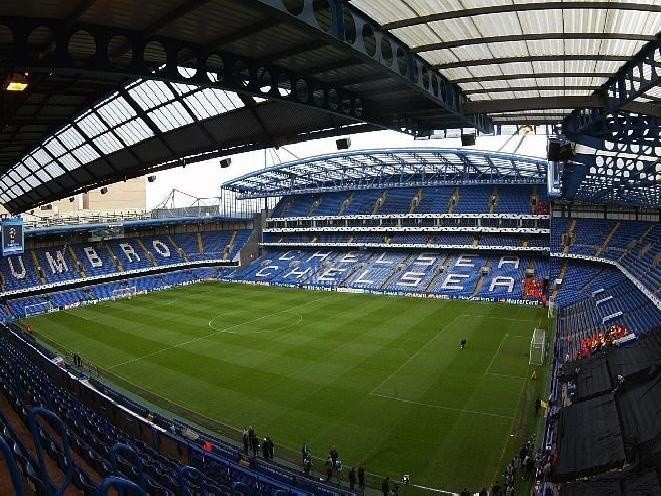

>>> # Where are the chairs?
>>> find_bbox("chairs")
[0,361,256,496]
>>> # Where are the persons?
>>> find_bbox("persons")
[460,336,467,348]
[461,488,470,496]
[534,395,541,416]
[480,489,489,496]
[504,432,551,496]
[243,426,401,496]
[73,355,81,367]
[561,325,629,407]
[203,438,213,463]
[492,481,503,496]
[146,412,154,423]
